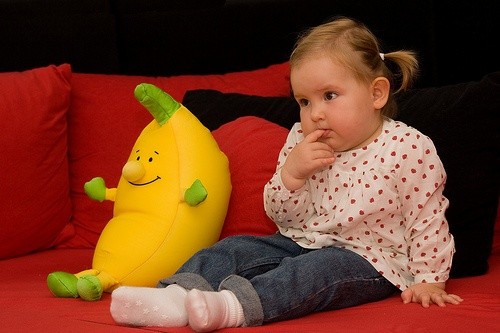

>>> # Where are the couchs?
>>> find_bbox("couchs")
[0,63,500,333]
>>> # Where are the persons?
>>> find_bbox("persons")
[110,20,463,332]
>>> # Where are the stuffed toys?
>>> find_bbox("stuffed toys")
[47,82,233,300]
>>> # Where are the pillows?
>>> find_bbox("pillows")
[0,62,70,261]
[180,89,304,240]
